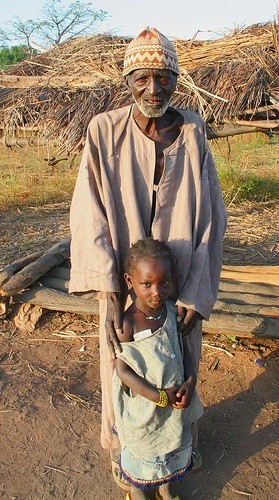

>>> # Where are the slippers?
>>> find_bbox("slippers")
[191,449,203,471]
[112,460,132,491]
[155,490,180,500]
[126,491,150,500]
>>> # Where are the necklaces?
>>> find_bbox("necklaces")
[133,302,165,321]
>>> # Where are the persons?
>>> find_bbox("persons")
[112,236,196,500]
[70,25,227,500]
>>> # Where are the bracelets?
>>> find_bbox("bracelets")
[154,389,169,407]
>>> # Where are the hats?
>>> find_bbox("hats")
[122,25,180,77]
